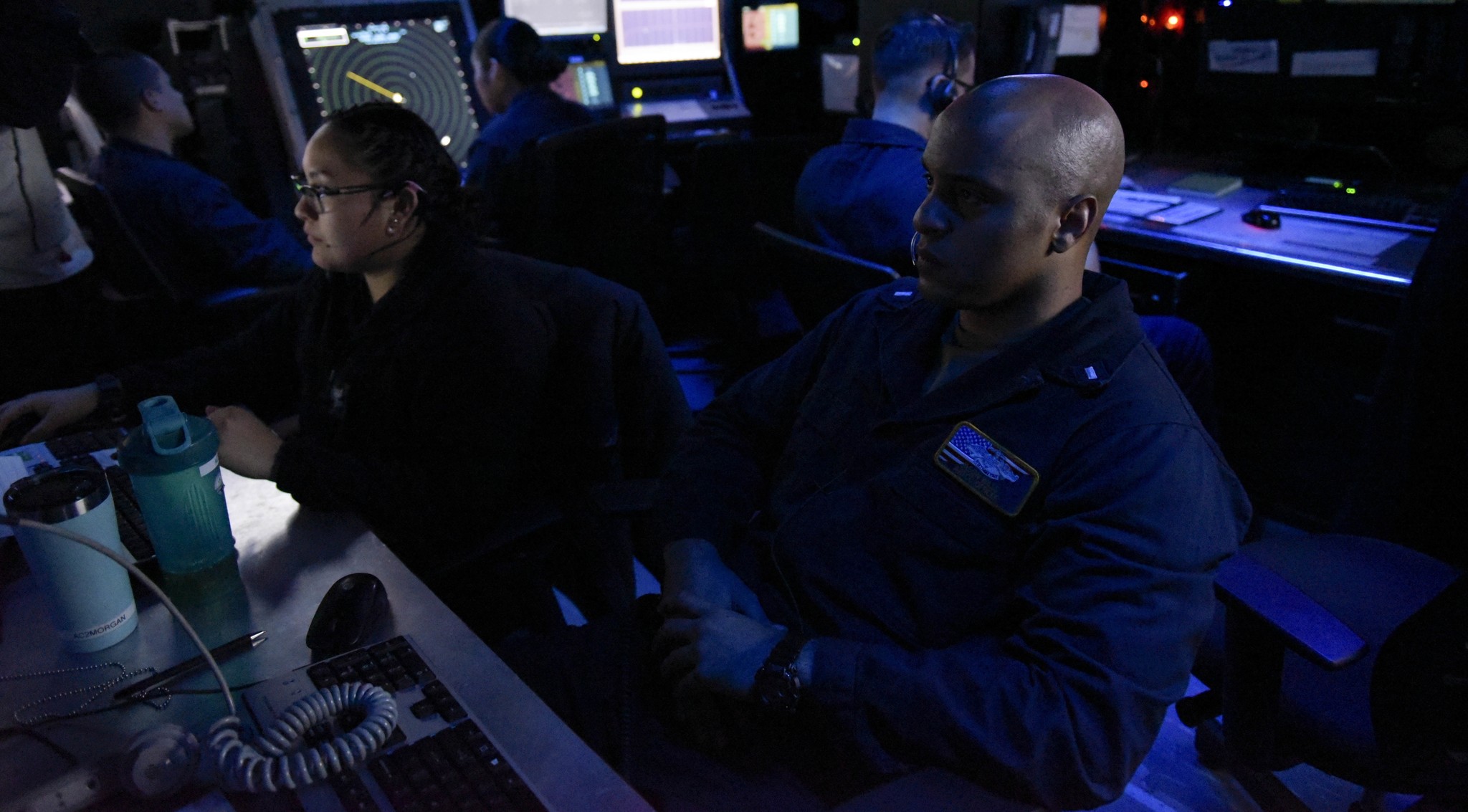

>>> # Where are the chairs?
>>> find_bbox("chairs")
[467,244,693,489]
[1125,307,1457,774]
[51,160,299,323]
[472,113,668,254]
[743,213,906,344]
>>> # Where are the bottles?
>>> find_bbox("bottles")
[117,396,236,575]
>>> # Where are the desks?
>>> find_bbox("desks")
[1097,149,1441,294]
[0,405,664,812]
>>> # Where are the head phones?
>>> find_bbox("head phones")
[925,12,961,109]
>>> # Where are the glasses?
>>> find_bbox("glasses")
[291,175,429,213]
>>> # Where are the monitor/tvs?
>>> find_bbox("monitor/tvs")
[500,0,610,44]
[605,1,726,77]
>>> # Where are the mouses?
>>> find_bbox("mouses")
[305,572,385,653]
[1240,209,1282,230]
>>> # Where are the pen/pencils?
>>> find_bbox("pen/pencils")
[1127,196,1175,207]
[113,628,265,701]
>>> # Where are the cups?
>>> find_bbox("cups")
[3,464,140,653]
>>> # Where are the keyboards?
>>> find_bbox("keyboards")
[245,632,558,812]
[40,425,157,565]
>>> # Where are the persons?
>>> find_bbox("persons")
[793,5,1217,433]
[85,52,323,364]
[0,103,568,647]
[0,2,106,400]
[500,73,1262,812]
[461,16,593,252]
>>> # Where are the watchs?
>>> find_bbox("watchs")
[751,630,812,715]
